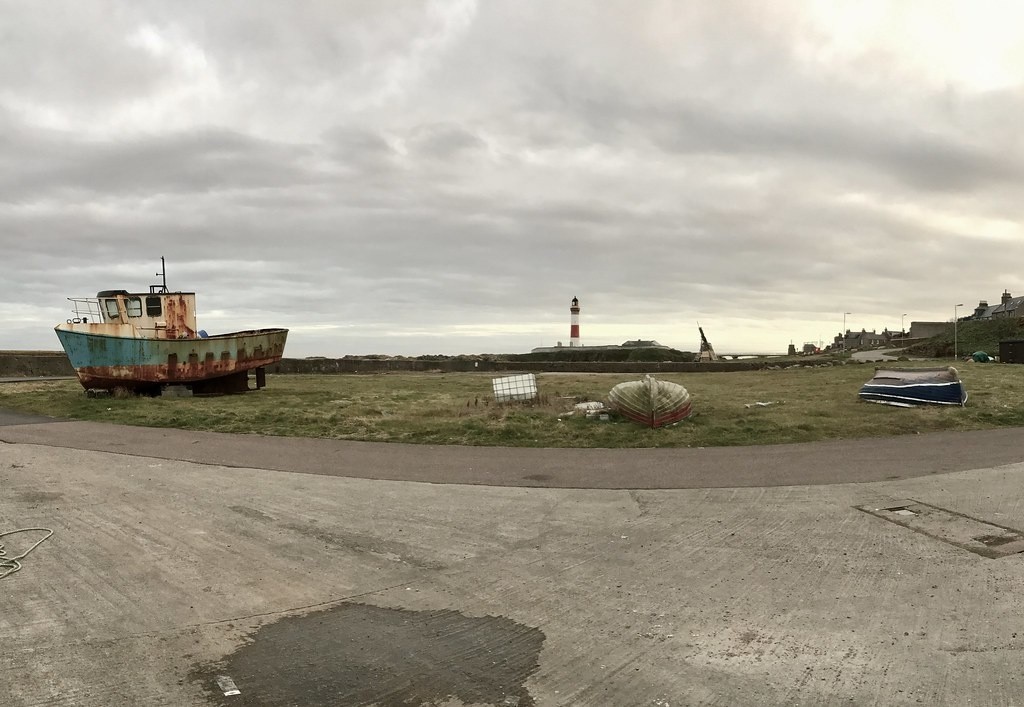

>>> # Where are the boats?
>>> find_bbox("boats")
[607,374,693,430]
[858,365,968,410]
[54,255,293,400]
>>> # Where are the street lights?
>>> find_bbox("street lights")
[843,313,852,356]
[902,313,907,347]
[955,304,964,361]
